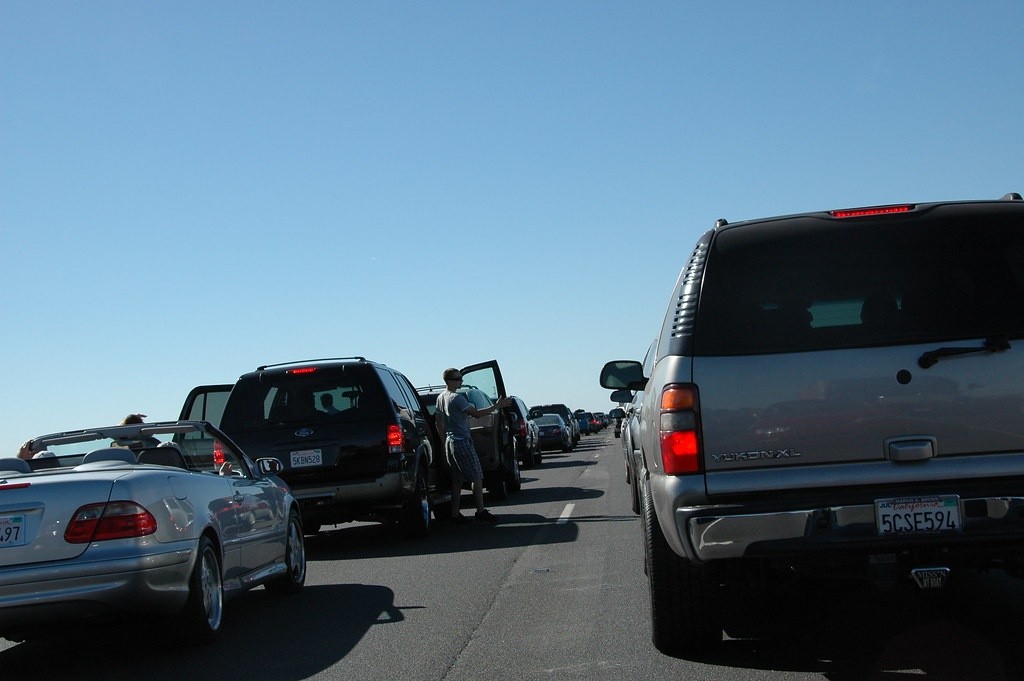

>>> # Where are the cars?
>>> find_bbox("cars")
[414,384,499,417]
[527,404,626,452]
[529,414,575,455]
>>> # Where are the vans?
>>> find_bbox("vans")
[167,354,519,536]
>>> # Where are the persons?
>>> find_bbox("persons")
[267,460,277,471]
[157,441,233,475]
[118,414,162,448]
[17,439,60,466]
[296,393,326,417]
[435,368,512,524]
[320,393,340,416]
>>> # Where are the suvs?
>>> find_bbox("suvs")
[597,193,1024,658]
[488,397,546,470]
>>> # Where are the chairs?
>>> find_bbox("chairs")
[0,459,30,473]
[82,442,196,471]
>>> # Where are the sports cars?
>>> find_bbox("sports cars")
[0,421,307,639]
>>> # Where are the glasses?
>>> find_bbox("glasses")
[448,377,462,382]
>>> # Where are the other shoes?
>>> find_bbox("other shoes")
[474,509,507,526]
[453,512,473,524]
